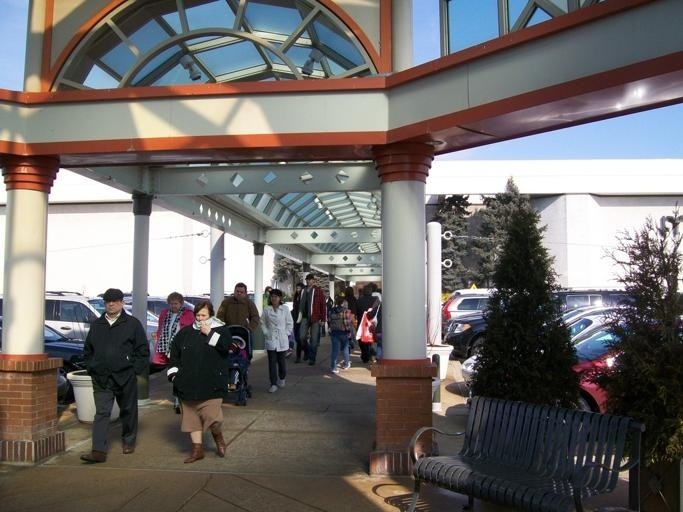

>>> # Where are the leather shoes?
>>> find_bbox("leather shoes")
[122,441,133,453]
[78,449,107,463]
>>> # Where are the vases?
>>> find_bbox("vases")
[67,369,120,424]
[426,343,454,380]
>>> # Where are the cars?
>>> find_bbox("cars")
[0,283,255,406]
[441,284,681,446]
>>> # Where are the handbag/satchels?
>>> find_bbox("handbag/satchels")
[356,311,377,343]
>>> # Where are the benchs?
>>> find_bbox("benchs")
[408,396,645,512]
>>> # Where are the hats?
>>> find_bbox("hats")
[102,288,124,300]
[304,274,313,280]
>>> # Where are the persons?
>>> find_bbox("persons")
[216,274,381,388]
[155,293,233,463]
[80,289,149,464]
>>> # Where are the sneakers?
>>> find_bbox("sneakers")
[294,354,369,375]
[268,385,278,393]
[278,378,285,388]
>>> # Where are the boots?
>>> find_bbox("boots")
[183,443,205,464]
[213,431,227,458]
